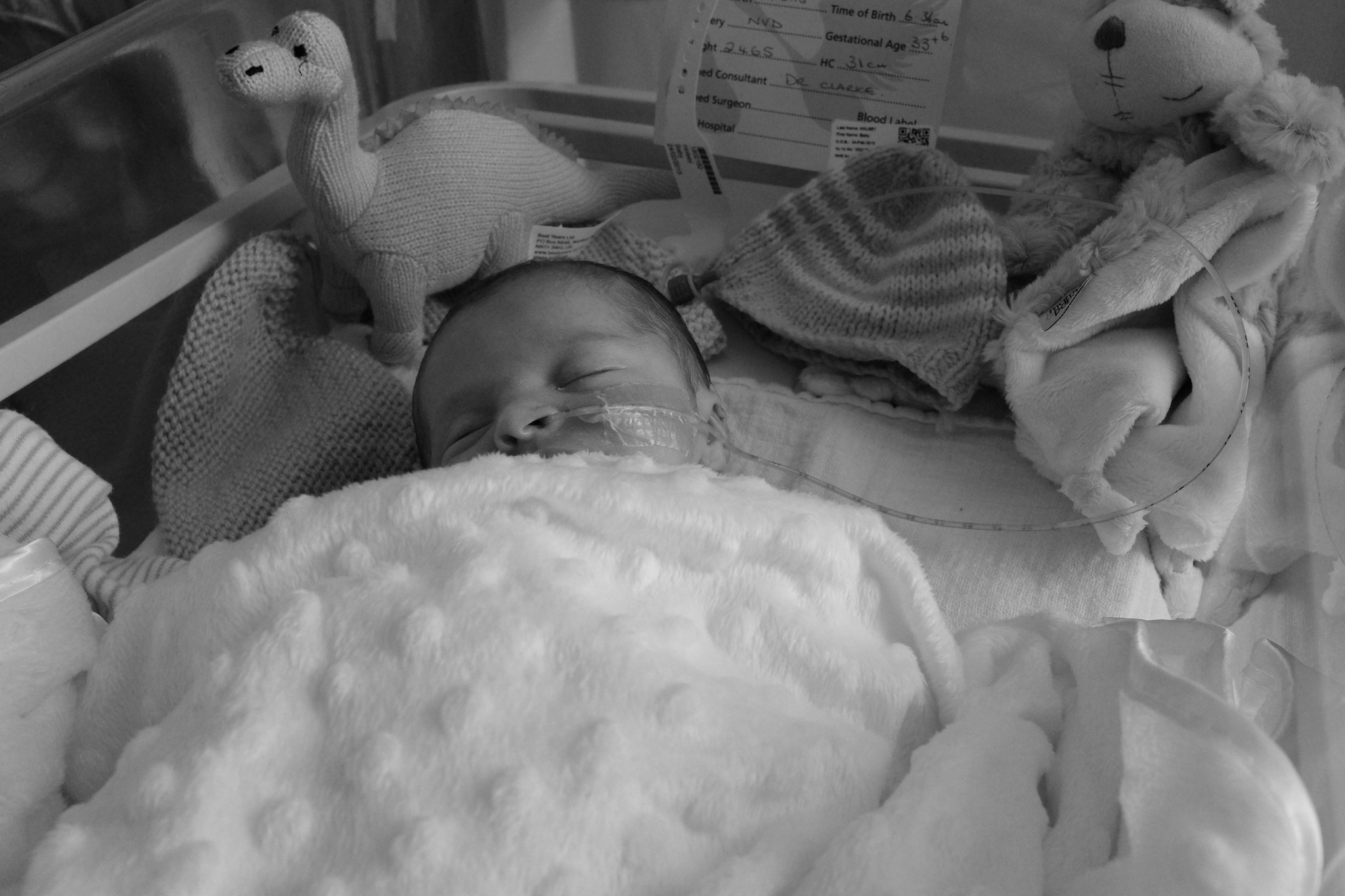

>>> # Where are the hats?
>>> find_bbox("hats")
[696,149,1006,411]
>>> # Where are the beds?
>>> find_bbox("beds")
[0,0,1345,896]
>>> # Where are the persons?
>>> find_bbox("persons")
[410,257,731,473]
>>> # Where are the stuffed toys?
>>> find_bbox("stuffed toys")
[216,9,687,367]
[1000,0,1345,283]
